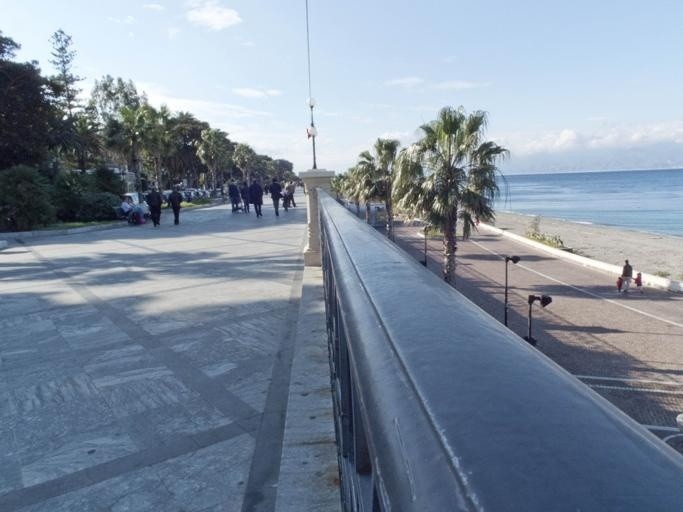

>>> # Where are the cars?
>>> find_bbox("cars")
[119,187,222,207]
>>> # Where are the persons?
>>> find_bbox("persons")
[269,177,296,216]
[248,180,263,217]
[147,189,162,227]
[633,273,642,292]
[229,179,239,213]
[167,188,183,224]
[616,277,623,292]
[241,182,251,213]
[120,196,146,224]
[621,260,632,291]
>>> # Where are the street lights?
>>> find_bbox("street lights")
[422,225,428,267]
[504,256,520,327]
[307,97,317,169]
[527,293,551,344]
